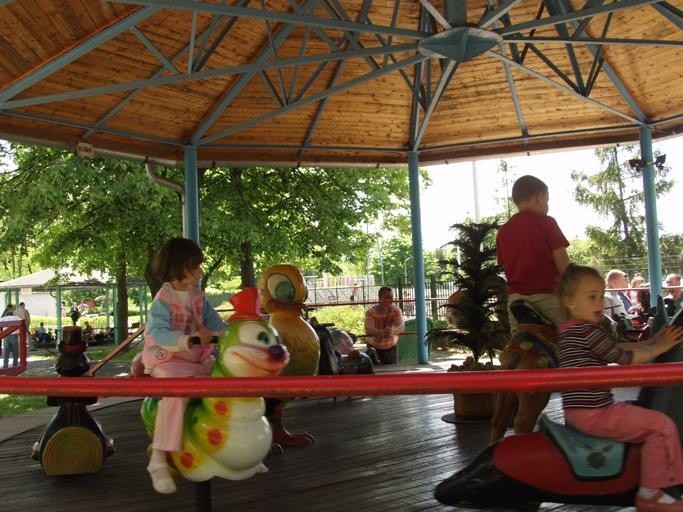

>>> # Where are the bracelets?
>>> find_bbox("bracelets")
[643,344,655,364]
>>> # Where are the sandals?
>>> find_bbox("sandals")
[147,462,178,494]
[635,490,682,512]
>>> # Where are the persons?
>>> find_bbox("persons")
[364,287,405,364]
[556,267,683,512]
[628,273,645,306]
[88,298,93,313]
[496,176,570,335]
[141,237,231,494]
[1,305,14,318]
[39,322,46,335]
[95,295,106,313]
[14,302,31,354]
[71,302,80,326]
[404,291,414,316]
[61,300,66,318]
[628,282,650,314]
[104,327,110,337]
[628,306,644,328]
[663,273,683,312]
[350,283,363,301]
[0,309,22,368]
[84,321,92,348]
[79,301,89,318]
[602,269,632,321]
